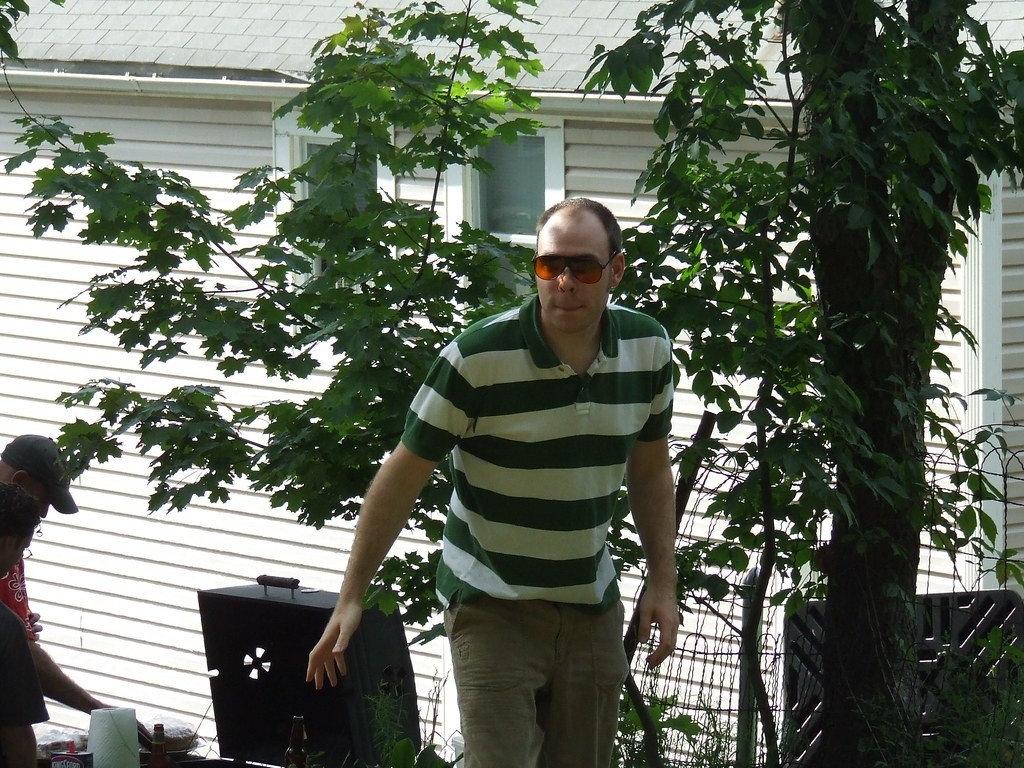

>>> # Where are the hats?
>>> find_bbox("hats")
[2,433,78,514]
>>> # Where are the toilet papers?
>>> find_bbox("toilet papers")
[86,706,140,768]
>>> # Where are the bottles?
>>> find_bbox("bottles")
[285,716,307,768]
[146,724,172,768]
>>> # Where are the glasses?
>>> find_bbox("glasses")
[531,250,619,285]
[20,544,33,559]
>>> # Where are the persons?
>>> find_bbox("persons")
[0,433,150,768]
[306,198,681,768]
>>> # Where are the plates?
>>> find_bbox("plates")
[139,748,197,758]
